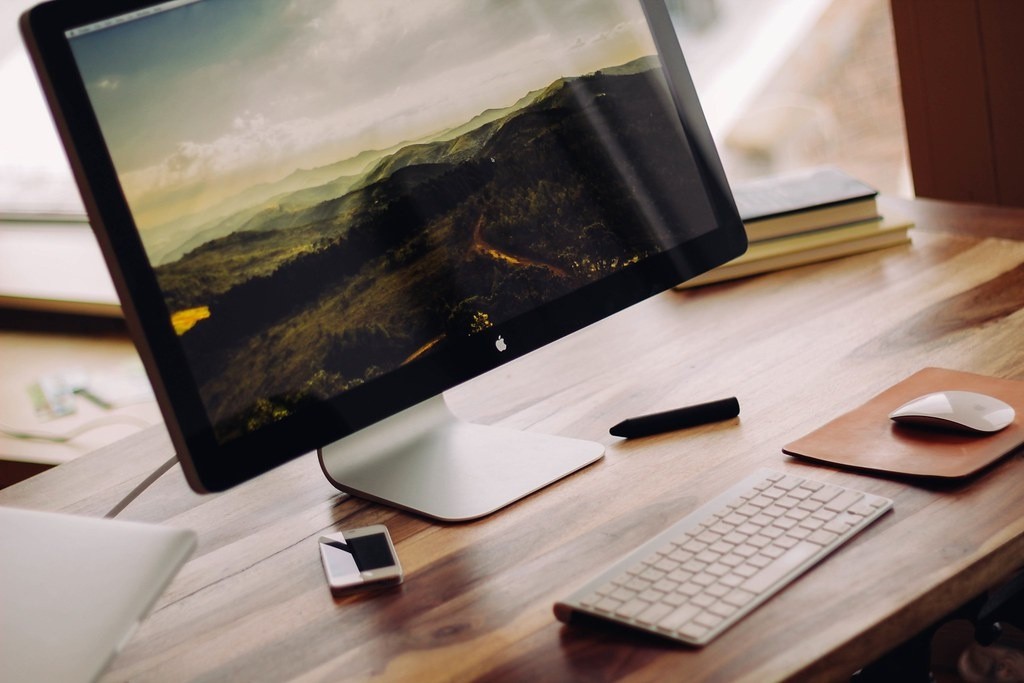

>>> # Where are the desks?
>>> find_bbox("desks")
[1,193,1024,683]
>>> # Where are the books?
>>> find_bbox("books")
[669,168,916,289]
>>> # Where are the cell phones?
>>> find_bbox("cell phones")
[320,524,403,597]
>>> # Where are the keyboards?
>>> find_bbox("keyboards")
[554,468,893,649]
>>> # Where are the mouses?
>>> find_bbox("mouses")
[889,390,1016,434]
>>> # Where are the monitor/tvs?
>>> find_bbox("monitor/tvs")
[22,0,749,523]
